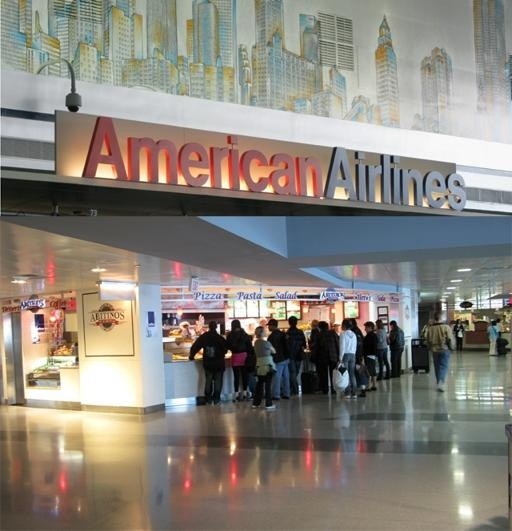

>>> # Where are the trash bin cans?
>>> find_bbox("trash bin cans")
[411,339,430,374]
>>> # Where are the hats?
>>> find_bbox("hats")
[266,319,278,326]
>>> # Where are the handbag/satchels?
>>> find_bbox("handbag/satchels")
[332,367,349,393]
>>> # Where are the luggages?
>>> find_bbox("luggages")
[301,353,320,394]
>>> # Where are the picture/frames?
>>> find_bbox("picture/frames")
[377,304,390,334]
[81,290,136,358]
[226,300,303,322]
[342,300,360,321]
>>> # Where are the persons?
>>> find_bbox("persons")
[421,318,433,351]
[187,314,408,410]
[454,318,466,352]
[500,314,510,324]
[486,320,499,356]
[426,309,454,392]
[496,317,504,332]
